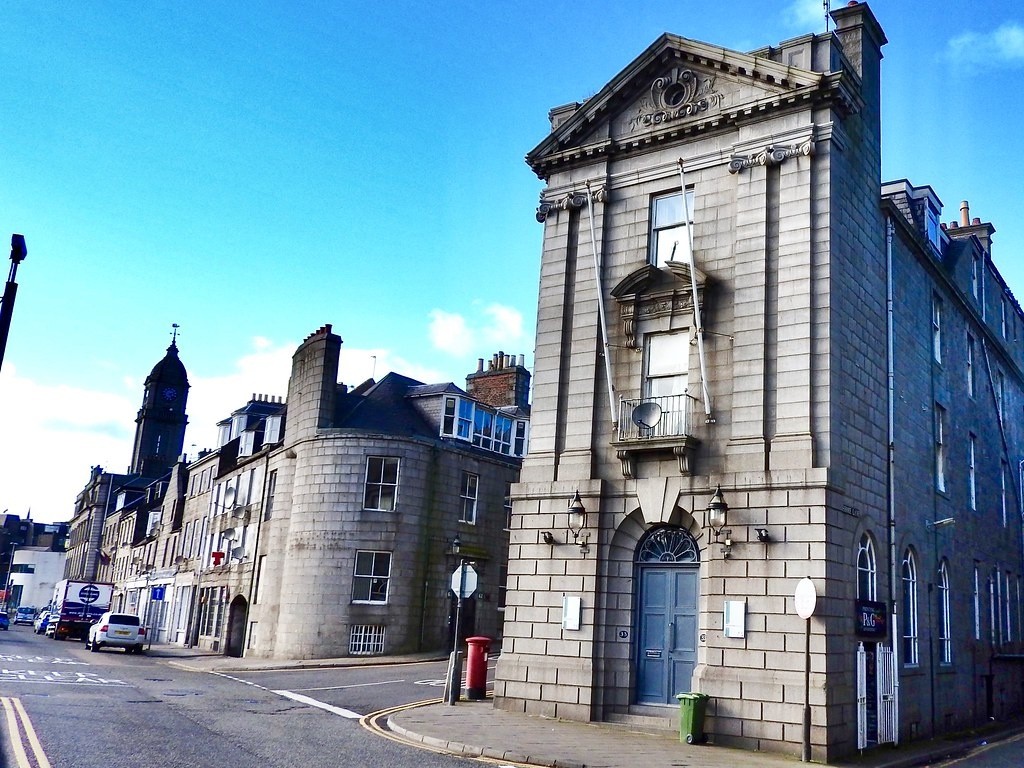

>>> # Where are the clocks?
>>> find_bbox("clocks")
[162,387,177,401]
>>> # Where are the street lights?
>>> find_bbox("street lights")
[1,542,18,611]
[446,534,461,656]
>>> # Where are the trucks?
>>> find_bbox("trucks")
[45,579,115,642]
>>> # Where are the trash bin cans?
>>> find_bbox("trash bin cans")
[676,692,710,745]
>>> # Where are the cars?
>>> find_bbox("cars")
[14,606,37,626]
[34,611,51,633]
[35,613,51,634]
[0,612,10,631]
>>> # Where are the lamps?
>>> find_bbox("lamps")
[567,489,586,547]
[924,518,957,533]
[707,483,730,544]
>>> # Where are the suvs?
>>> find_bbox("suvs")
[85,610,145,655]
